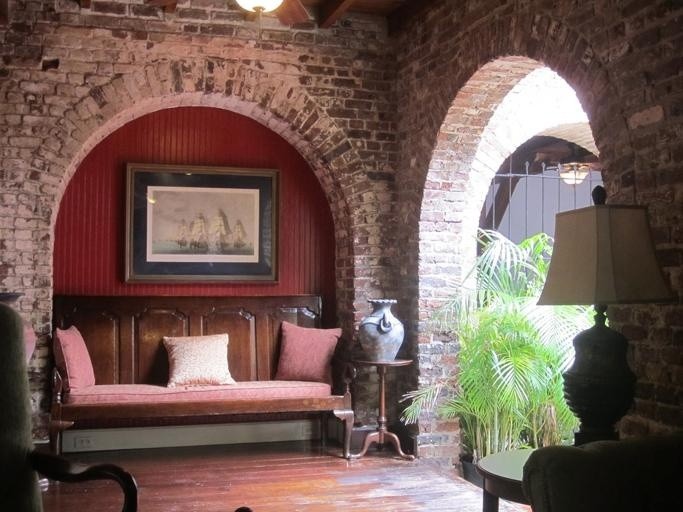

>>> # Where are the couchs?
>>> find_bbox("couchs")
[52,295,358,461]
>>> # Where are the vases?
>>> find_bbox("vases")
[358,299,404,362]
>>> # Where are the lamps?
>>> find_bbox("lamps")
[536,186,680,448]
[556,162,592,185]
[236,0,284,12]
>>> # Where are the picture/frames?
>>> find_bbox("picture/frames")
[125,162,280,285]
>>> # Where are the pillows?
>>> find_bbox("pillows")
[272,321,343,390]
[53,325,95,396]
[163,333,237,388]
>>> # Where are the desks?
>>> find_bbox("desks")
[349,359,415,462]
[475,448,538,512]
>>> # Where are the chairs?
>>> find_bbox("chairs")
[0,302,138,512]
[520,431,682,512]
